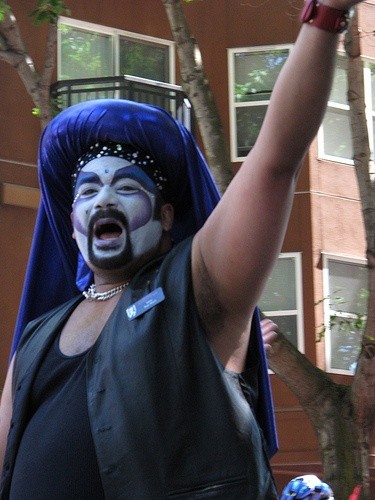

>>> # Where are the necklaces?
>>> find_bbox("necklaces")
[82,282,128,302]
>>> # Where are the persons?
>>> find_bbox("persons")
[0,0,365,499]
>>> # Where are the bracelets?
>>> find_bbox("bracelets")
[300,0,349,34]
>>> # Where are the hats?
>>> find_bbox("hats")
[280,475,334,500]
[8,99,279,461]
[368,454,375,469]
